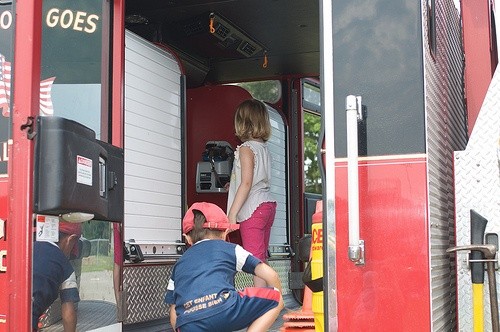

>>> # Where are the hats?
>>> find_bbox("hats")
[33,216,81,257]
[182,202,240,234]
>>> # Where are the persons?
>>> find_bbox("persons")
[226,99,276,286]
[33,223,80,332]
[163,202,284,332]
